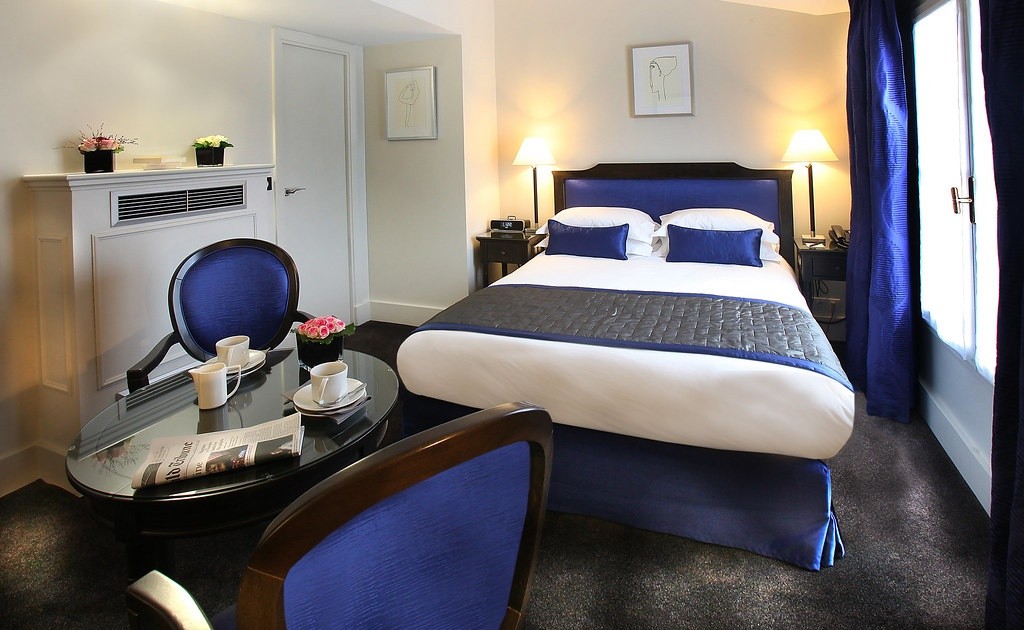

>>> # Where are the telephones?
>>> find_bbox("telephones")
[829,225,850,248]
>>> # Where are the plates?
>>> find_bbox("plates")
[284,407,366,438]
[200,349,266,380]
[227,369,267,396]
[292,379,367,417]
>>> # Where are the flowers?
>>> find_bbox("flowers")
[192,134,234,149]
[50,123,140,154]
[290,312,356,346]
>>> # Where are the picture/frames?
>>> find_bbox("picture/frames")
[382,66,437,140]
[631,43,692,115]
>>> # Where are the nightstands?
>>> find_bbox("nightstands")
[476,229,546,287]
[795,238,848,311]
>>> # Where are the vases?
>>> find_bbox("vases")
[83,150,113,172]
[194,148,223,166]
[295,332,343,373]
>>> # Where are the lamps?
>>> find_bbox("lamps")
[782,130,839,244]
[512,136,563,234]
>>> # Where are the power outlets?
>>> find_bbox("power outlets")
[811,297,842,314]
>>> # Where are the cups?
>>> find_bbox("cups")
[228,392,252,412]
[216,335,250,371]
[310,361,348,405]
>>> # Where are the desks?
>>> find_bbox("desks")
[65,347,401,573]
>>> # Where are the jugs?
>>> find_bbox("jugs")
[188,363,241,409]
[196,403,244,435]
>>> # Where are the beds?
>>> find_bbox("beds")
[394,127,856,571]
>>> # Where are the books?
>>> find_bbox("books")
[133,155,186,170]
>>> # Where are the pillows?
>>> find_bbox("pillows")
[531,206,782,268]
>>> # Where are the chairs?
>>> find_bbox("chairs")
[127,238,317,396]
[125,402,551,630]
